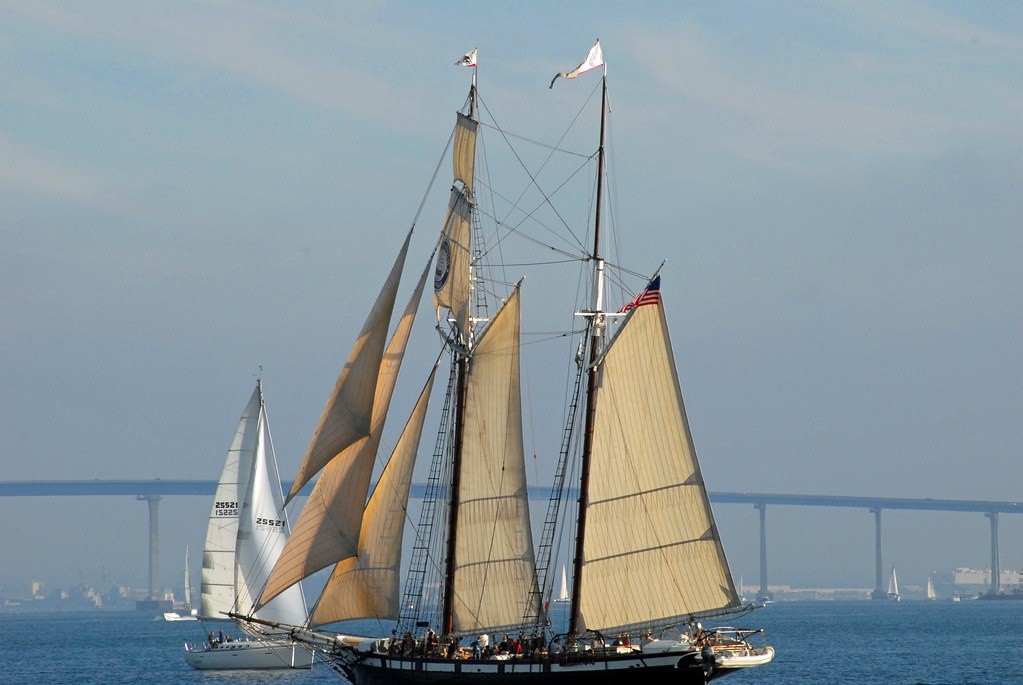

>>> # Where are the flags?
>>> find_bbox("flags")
[617,274,661,313]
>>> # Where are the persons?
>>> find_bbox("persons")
[388,628,399,652]
[208,631,217,648]
[212,630,227,647]
[470,630,543,658]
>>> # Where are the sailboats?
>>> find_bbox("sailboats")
[217,33,775,685]
[887,563,901,602]
[952,570,960,602]
[927,577,937,600]
[163,545,197,621]
[183,364,316,670]
[737,572,776,605]
